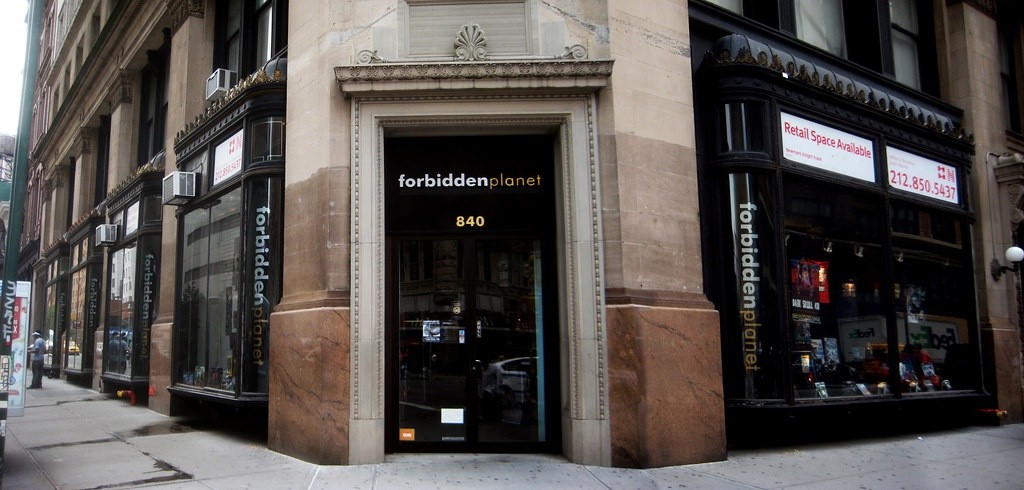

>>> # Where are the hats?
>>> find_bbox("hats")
[32,331,40,336]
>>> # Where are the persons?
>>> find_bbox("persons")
[28,331,46,388]
[109,331,128,375]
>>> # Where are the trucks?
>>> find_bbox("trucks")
[838,312,969,365]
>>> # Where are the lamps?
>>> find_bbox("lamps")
[784,233,790,246]
[895,251,903,262]
[991,246,1023,280]
[823,240,833,252]
[942,256,950,266]
[854,244,864,257]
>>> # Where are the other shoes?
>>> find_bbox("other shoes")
[27,384,35,389]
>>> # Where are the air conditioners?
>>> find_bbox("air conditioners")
[95,223,117,246]
[206,68,235,101]
[161,171,195,205]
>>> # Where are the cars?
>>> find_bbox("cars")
[481,357,538,410]
[793,357,857,387]
[64,341,80,356]
[96,342,103,359]
[864,343,935,379]
